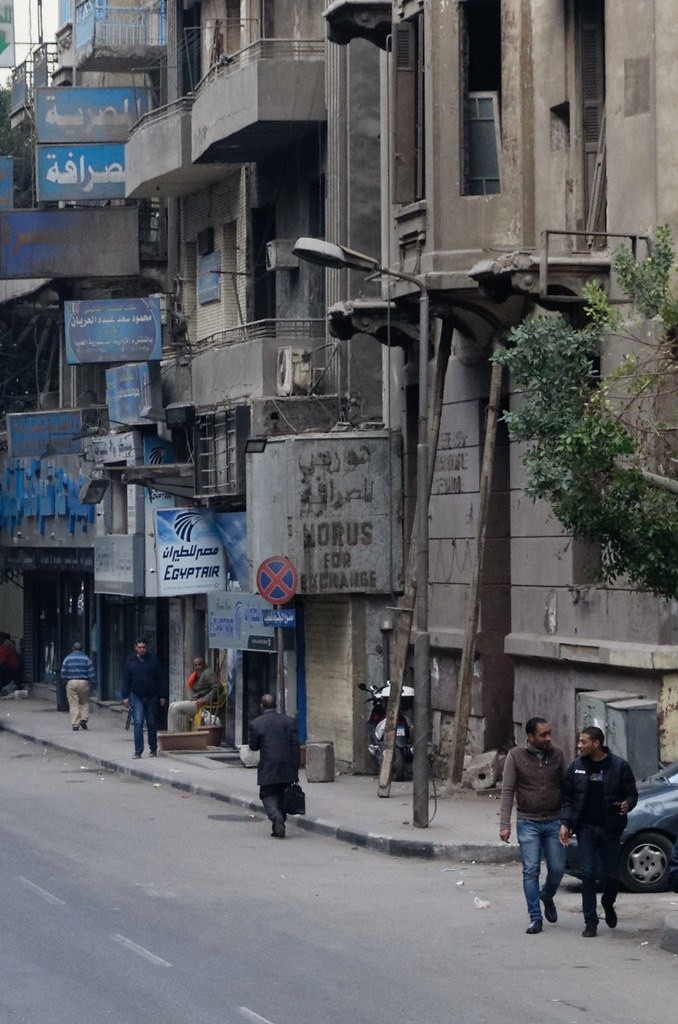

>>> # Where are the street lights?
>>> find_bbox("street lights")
[292,237,430,830]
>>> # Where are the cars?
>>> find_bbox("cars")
[564,758,678,893]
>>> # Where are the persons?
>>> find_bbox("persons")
[499,716,639,937]
[249,695,300,838]
[167,657,224,731]
[0,632,24,689]
[121,636,167,759]
[61,642,95,730]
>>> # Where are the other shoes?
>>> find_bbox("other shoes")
[79,720,87,729]
[133,751,141,759]
[73,726,78,731]
[526,919,542,934]
[601,898,618,929]
[270,815,287,838]
[540,890,557,923]
[148,751,157,757]
[583,917,599,938]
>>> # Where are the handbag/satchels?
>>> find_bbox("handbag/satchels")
[281,780,306,816]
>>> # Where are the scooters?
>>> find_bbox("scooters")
[357,682,415,783]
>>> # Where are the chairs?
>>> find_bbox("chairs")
[191,678,232,732]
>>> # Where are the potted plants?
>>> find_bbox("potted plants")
[194,649,229,745]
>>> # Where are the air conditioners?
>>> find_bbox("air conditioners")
[277,345,312,396]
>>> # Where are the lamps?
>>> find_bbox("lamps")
[78,467,108,505]
[244,433,274,454]
